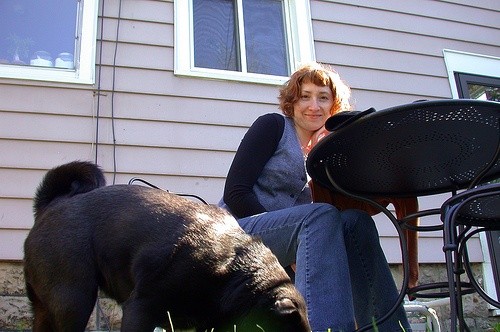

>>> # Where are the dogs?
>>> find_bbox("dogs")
[23,159,312,332]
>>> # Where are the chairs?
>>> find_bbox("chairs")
[440,183,500,332]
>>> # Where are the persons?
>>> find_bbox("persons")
[218,62,413,332]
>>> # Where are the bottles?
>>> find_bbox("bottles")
[55,52,74,69]
[30,51,54,67]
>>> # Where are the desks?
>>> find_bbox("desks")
[306,99,500,332]
